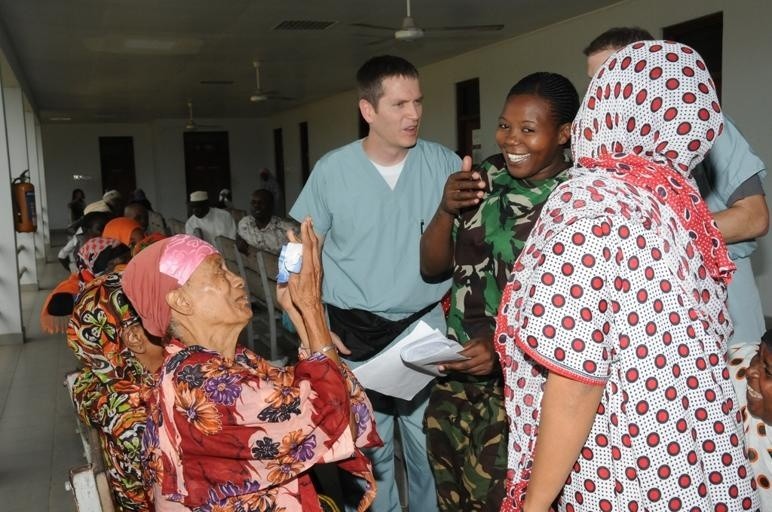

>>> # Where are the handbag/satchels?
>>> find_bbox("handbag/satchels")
[326,306,406,362]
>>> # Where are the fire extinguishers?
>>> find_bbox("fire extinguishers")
[11,170,38,233]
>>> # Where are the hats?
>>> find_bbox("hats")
[190,191,208,202]
[103,190,121,204]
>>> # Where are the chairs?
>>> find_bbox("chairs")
[63,371,116,512]
[148,210,282,360]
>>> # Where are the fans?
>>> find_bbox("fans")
[226,59,301,103]
[348,0,505,48]
[161,97,228,132]
